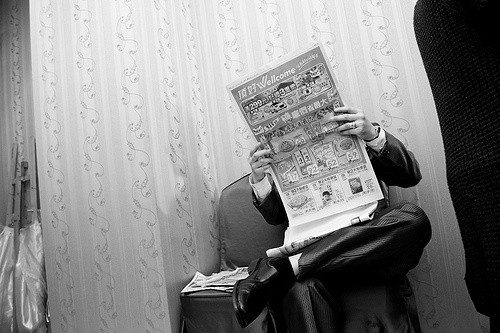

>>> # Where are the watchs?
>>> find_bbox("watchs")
[364,125,380,142]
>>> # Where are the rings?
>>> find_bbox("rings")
[352,122,356,129]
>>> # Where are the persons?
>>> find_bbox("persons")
[233,107,432,333]
[321,191,332,202]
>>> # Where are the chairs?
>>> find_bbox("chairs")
[413,0,500,333]
[178,169,420,333]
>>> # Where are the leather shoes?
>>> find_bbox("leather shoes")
[232,257,282,327]
